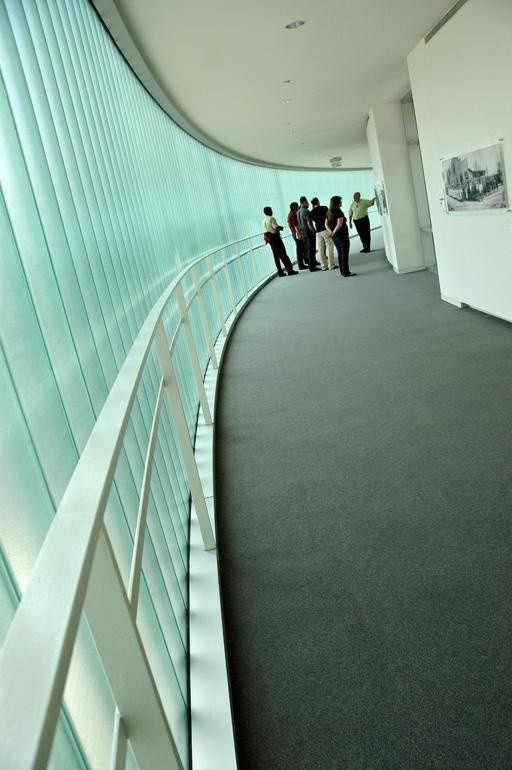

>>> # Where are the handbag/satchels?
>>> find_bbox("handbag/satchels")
[263,232,275,242]
[295,232,302,240]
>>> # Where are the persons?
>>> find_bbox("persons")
[288,203,309,269]
[296,196,322,272]
[309,198,339,271]
[324,196,357,277]
[263,207,299,277]
[349,192,376,253]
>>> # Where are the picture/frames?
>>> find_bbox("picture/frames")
[439,137,512,216]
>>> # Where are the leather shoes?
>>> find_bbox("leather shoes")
[360,248,370,253]
[279,261,357,278]
